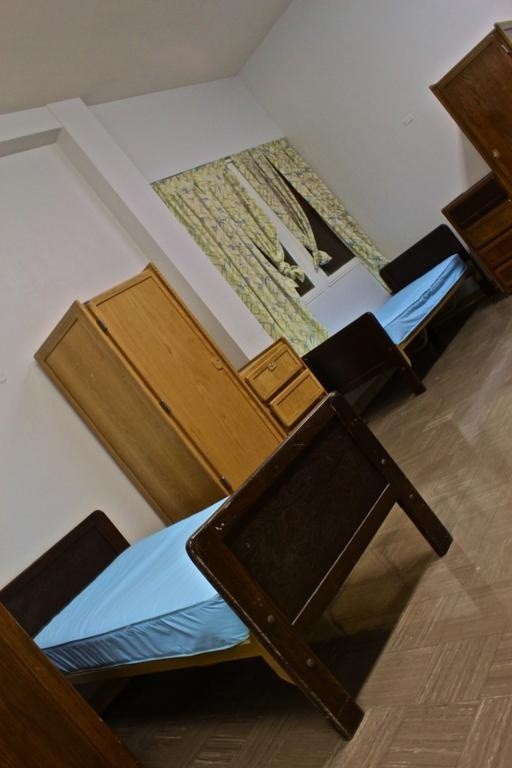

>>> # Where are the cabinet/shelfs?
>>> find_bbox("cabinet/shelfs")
[428,21,512,189]
[32,265,293,526]
[443,170,512,300]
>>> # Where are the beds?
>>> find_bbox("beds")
[301,226,493,393]
[1,387,455,738]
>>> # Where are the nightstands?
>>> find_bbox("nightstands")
[241,339,328,430]
